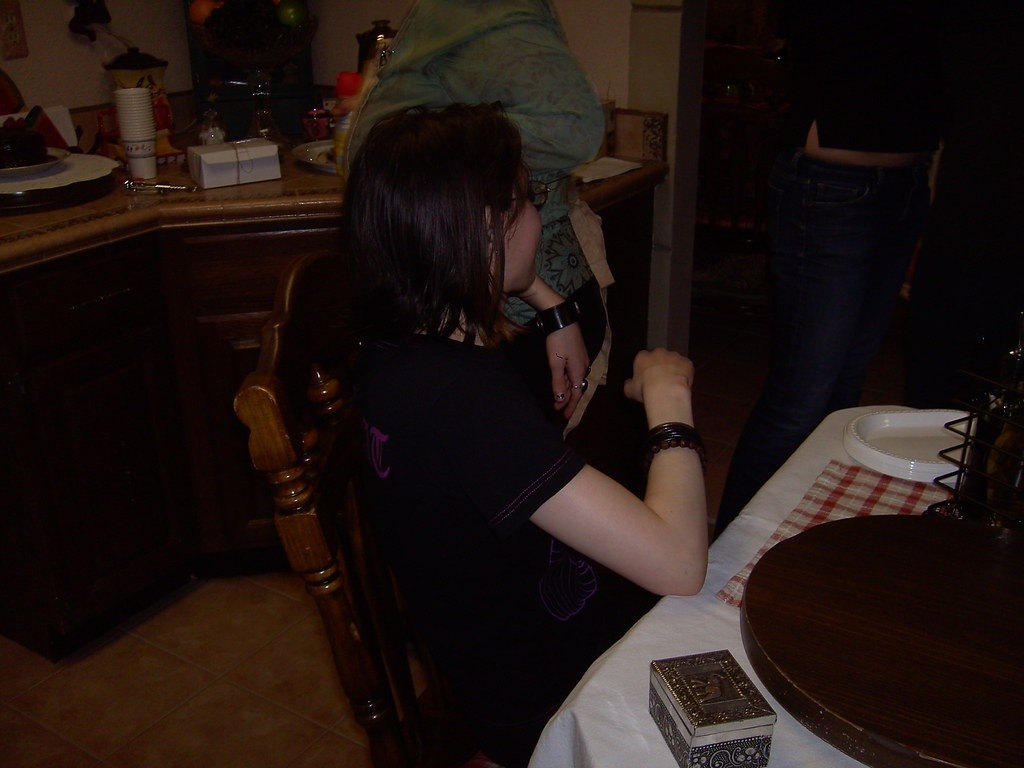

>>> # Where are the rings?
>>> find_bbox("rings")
[571,378,588,395]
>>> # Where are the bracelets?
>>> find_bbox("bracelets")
[643,423,708,477]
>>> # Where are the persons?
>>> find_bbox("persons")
[713,1,941,555]
[336,103,710,768]
[333,0,618,442]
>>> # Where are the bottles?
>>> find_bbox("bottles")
[198,100,225,146]
[246,70,286,163]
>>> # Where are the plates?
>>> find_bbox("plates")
[842,409,974,483]
[292,139,342,173]
[0,146,71,177]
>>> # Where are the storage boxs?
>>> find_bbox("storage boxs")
[185,137,282,190]
[648,649,778,768]
[594,98,617,159]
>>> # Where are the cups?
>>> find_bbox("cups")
[112,87,157,180]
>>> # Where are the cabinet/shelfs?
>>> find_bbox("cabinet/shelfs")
[0,156,671,665]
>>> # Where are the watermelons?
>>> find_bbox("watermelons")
[22,105,72,149]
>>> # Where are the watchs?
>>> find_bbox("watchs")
[535,293,581,344]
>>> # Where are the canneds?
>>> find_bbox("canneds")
[334,117,351,175]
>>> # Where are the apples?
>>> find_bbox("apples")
[187,0,224,24]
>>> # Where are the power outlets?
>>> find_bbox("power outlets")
[0,0,29,63]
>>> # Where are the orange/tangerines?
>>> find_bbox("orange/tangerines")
[276,0,309,26]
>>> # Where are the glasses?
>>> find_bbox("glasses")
[511,180,550,209]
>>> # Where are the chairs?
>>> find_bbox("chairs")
[227,248,503,768]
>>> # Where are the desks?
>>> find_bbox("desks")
[522,402,971,768]
